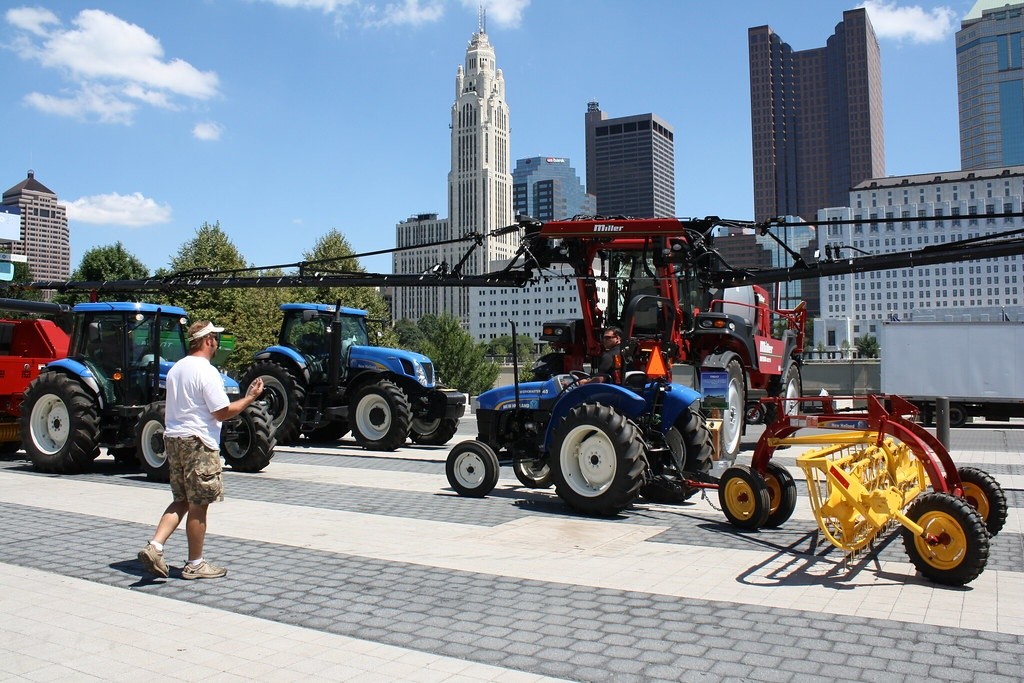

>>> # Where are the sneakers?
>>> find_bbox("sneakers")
[138,541,168,580]
[182,562,226,581]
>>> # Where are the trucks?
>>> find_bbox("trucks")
[878,318,1023,428]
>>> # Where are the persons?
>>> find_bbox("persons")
[579,326,622,386]
[137,321,265,581]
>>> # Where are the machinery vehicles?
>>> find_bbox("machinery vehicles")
[453,361,711,519]
[0,319,78,460]
[241,300,470,451]
[22,298,278,480]
[717,389,1008,587]
[519,214,804,429]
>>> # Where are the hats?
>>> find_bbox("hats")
[187,321,225,340]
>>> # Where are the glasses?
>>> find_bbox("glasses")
[603,336,617,340]
[212,335,220,340]
